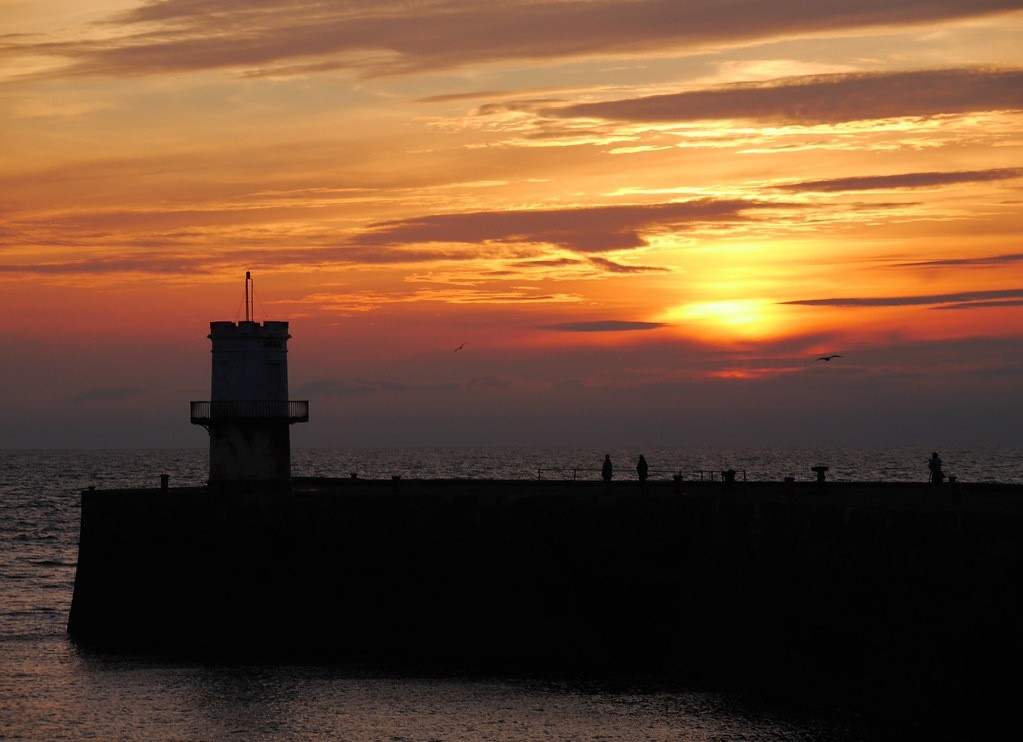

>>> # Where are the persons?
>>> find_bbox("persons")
[636,455,648,481]
[601,454,612,481]
[928,452,943,483]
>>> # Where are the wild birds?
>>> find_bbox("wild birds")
[455,344,464,351]
[816,355,841,362]
